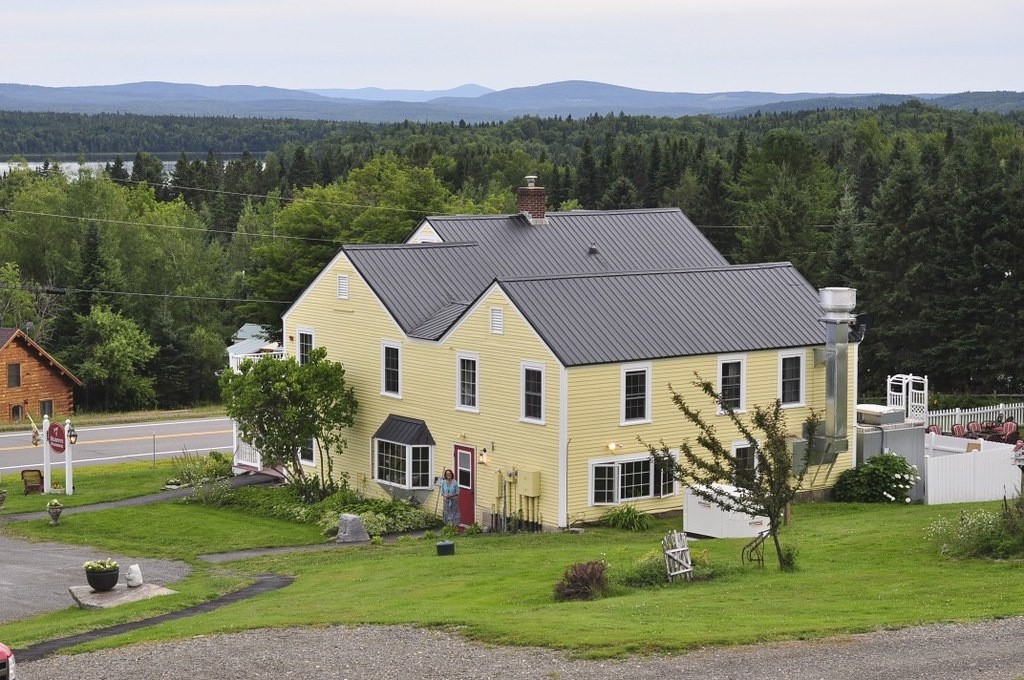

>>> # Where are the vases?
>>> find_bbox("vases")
[47,506,63,525]
[51,489,64,494]
[85,571,119,591]
[0,490,7,509]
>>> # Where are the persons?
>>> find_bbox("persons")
[441,470,460,526]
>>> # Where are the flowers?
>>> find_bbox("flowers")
[47,499,63,507]
[82,557,118,572]
[51,481,64,490]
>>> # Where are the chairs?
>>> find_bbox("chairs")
[928,421,1019,445]
[21,469,44,496]
